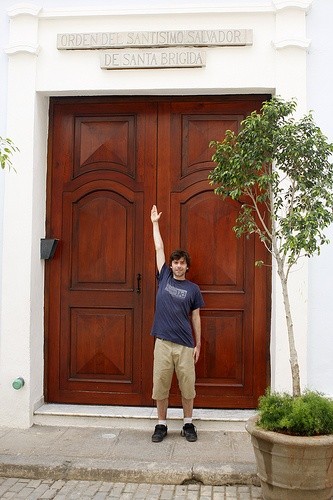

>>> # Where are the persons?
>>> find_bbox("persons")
[150,204,204,443]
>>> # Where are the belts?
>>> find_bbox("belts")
[155,336,168,341]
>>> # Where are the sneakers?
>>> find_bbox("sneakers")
[152,424,167,442]
[183,423,196,442]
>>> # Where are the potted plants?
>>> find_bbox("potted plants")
[245,388,332,500]
